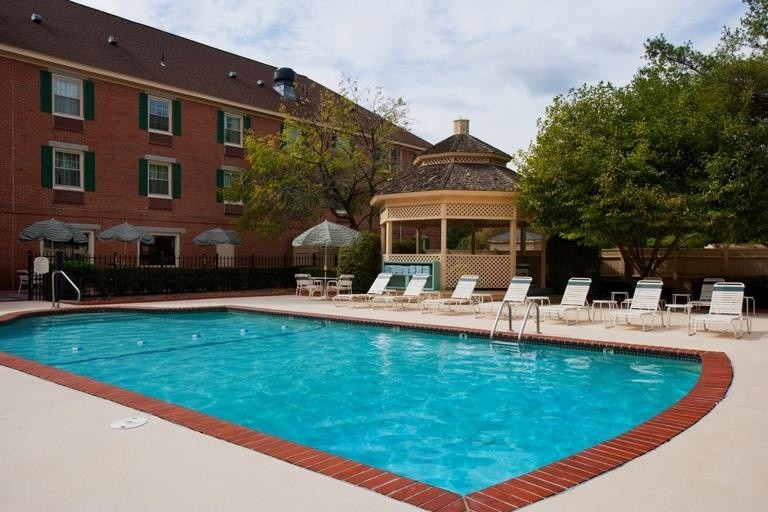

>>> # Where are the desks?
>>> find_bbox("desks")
[672,293,692,312]
[591,299,618,326]
[470,293,493,315]
[743,296,756,316]
[525,296,550,321]
[376,289,398,308]
[610,291,629,309]
[419,291,441,311]
[664,303,694,330]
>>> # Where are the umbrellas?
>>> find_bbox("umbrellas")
[293,219,362,296]
[192,226,244,255]
[18,218,88,263]
[96,223,155,253]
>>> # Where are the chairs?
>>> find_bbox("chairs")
[604,279,668,331]
[684,277,725,314]
[473,275,533,318]
[421,274,479,312]
[294,273,355,304]
[333,272,393,305]
[533,277,592,326]
[370,273,430,310]
[687,281,751,339]
[16,270,35,294]
[620,276,663,312]
